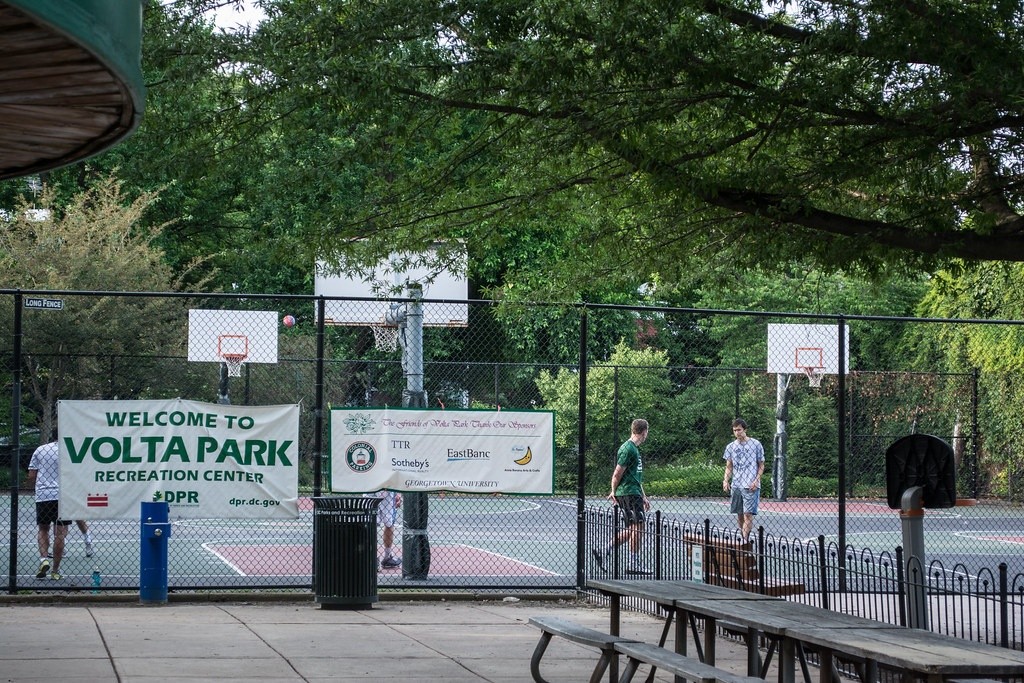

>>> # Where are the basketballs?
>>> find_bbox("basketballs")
[283,315,295,327]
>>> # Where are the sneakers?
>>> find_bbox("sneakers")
[84,540,93,556]
[593,546,608,572]
[625,562,654,575]
[48,547,68,558]
[50,571,60,580]
[36,558,51,577]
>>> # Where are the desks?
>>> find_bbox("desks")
[585,578,787,683]
[785,627,1024,683]
[676,599,911,683]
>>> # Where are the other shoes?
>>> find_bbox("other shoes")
[381,556,402,568]
[378,556,381,573]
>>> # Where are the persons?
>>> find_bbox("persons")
[722,420,764,539]
[363,491,401,570]
[594,419,654,573]
[46,432,94,557]
[29,426,72,580]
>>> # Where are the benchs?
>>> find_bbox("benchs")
[614,642,738,683]
[683,532,805,596]
[528,613,644,683]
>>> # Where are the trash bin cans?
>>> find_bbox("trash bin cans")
[308,494,385,611]
